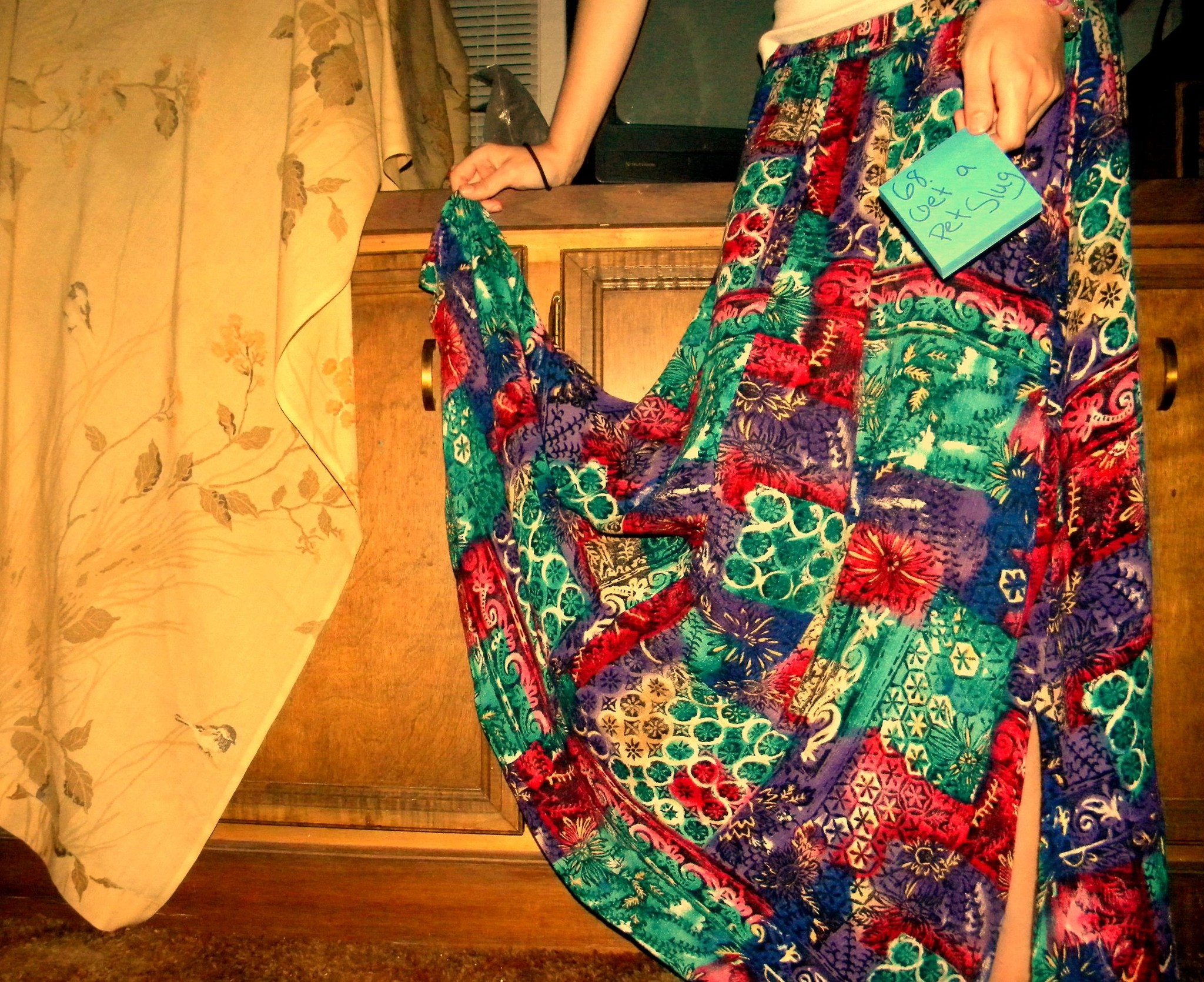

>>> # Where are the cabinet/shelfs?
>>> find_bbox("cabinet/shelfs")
[160,181,1203,948]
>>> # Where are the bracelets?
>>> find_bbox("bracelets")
[519,140,555,196]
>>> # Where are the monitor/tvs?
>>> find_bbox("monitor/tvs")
[586,0,776,184]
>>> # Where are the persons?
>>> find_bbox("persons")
[419,1,1187,982]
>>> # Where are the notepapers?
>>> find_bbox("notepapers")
[878,129,1043,281]
